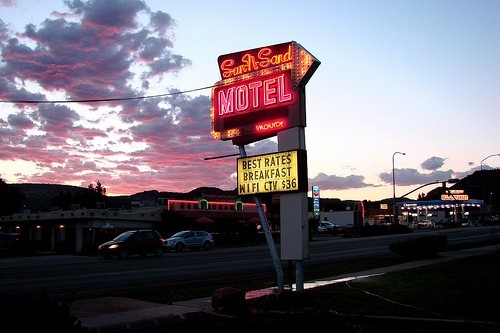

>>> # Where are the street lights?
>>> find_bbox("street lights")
[481,154,500,170]
[393,151,406,205]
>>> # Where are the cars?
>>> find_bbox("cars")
[317,220,354,233]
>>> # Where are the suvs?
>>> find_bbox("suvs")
[98,228,165,260]
[162,230,214,252]
[417,220,433,230]
